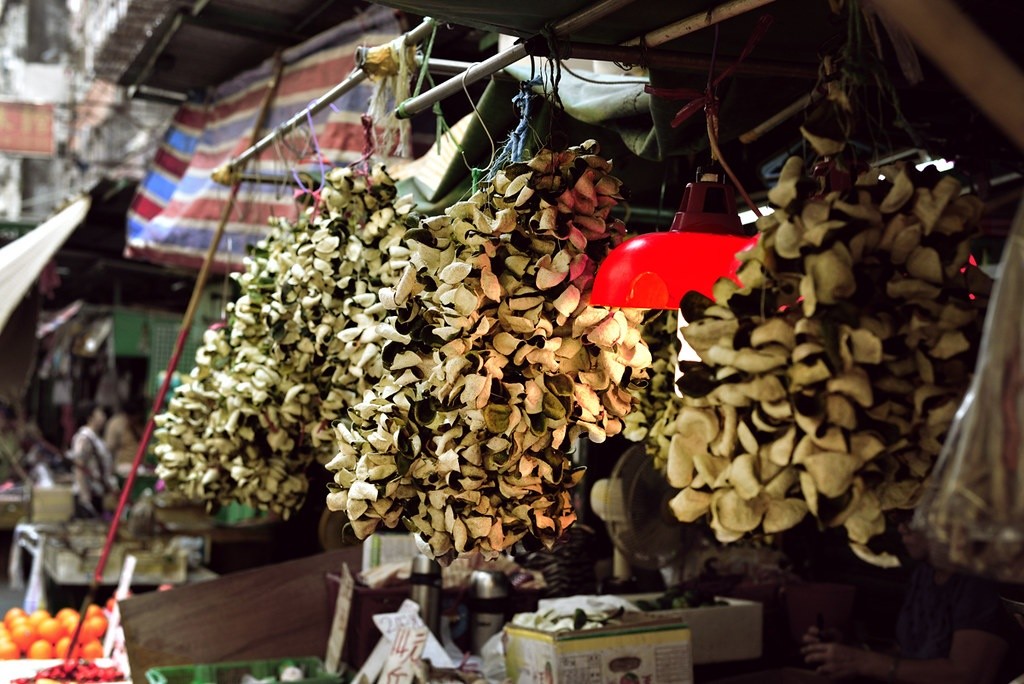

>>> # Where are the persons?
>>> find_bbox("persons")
[68,405,123,516]
[106,403,138,464]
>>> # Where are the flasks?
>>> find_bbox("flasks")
[471,570,513,657]
[411,554,441,639]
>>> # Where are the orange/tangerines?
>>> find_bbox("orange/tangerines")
[0,602,107,660]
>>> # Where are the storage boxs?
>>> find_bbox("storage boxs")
[503,594,762,684]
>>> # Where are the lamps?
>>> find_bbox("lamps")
[590,166,801,310]
